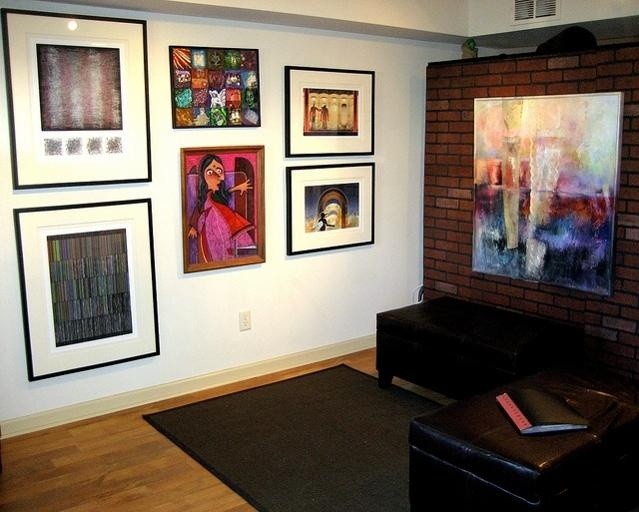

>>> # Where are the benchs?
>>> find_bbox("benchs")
[377,297,638,511]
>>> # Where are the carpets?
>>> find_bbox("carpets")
[142,365,446,511]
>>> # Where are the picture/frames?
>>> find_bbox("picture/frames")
[180,145,266,278]
[283,65,375,157]
[285,161,376,258]
[0,7,153,190]
[472,91,625,297]
[169,44,261,129]
[12,198,162,381]
